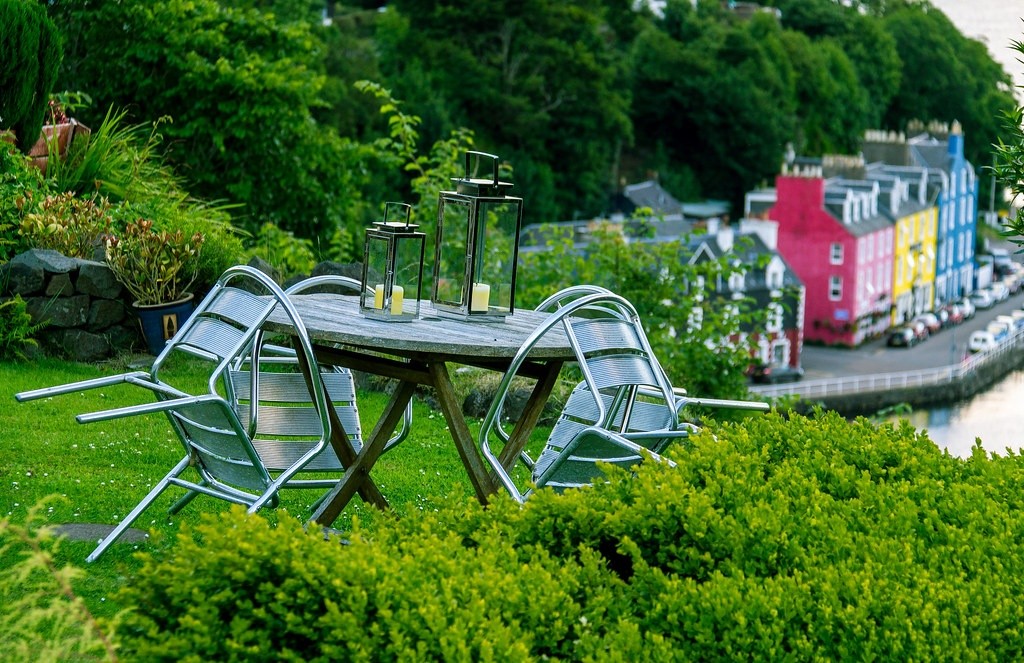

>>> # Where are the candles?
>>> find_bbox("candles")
[460,281,490,313]
[376,284,403,314]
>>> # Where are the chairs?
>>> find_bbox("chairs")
[15,265,768,565]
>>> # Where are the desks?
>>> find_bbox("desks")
[220,294,592,533]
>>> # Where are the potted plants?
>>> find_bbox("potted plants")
[104,221,205,355]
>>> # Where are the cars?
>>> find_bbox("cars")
[885,248,1024,356]
